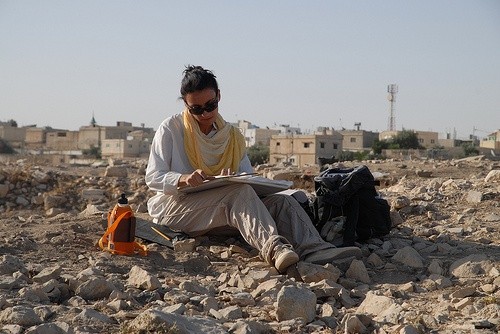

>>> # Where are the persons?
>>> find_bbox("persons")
[144,64,363,272]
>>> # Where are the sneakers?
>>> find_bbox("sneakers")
[275,246,300,273]
[303,246,363,266]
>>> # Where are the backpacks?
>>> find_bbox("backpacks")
[313,164,391,246]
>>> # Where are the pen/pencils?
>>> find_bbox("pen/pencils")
[151,226,171,240]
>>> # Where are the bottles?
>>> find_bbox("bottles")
[107,193,136,254]
